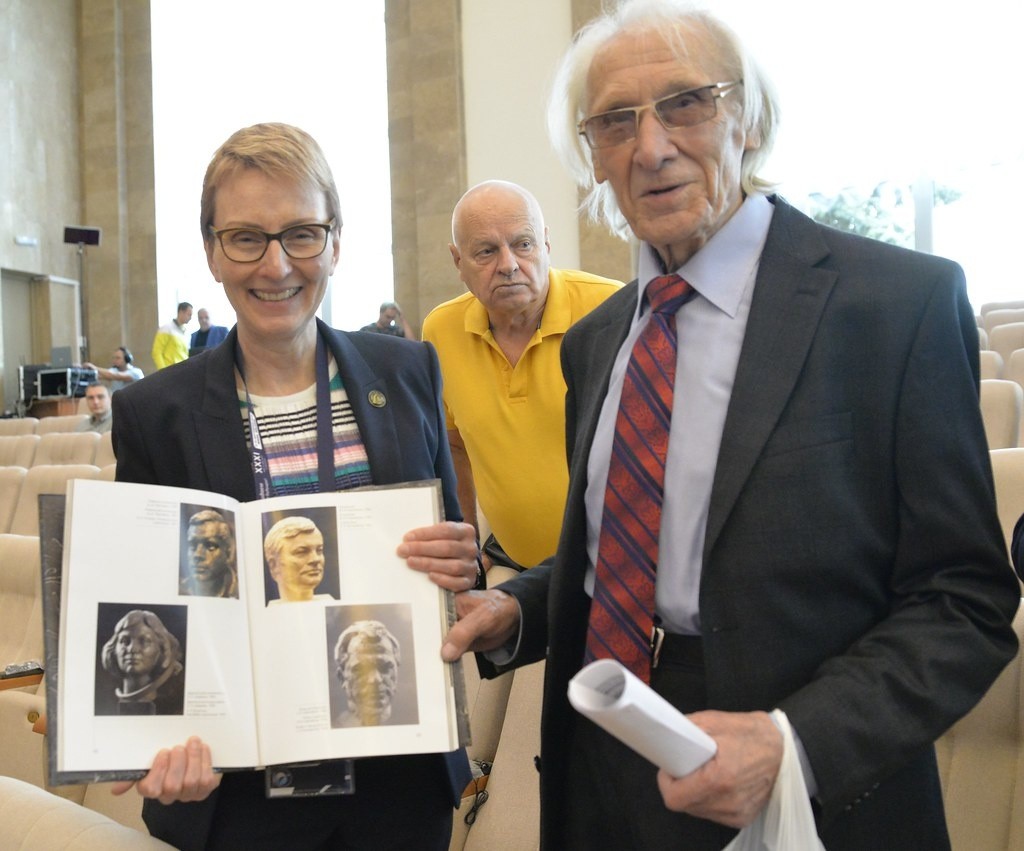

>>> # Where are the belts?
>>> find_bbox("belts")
[571,590,705,668]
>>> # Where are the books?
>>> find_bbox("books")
[37,477,473,790]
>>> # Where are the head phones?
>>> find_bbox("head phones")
[119,346,135,363]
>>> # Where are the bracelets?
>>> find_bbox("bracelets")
[473,561,481,588]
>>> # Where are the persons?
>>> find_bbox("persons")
[180,510,236,597]
[361,301,415,341]
[82,346,144,392]
[334,620,401,726]
[190,307,229,353]
[263,515,326,601]
[77,383,115,435]
[101,610,184,701]
[421,180,629,574]
[151,302,193,370]
[440,0,1021,851]
[109,122,492,851]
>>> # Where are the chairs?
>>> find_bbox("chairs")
[925,299,1024,851]
[0,415,548,851]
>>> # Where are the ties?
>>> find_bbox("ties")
[585,275,695,687]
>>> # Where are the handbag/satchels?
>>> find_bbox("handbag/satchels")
[719,709,827,851]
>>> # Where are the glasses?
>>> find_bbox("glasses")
[578,81,741,149]
[208,217,336,263]
